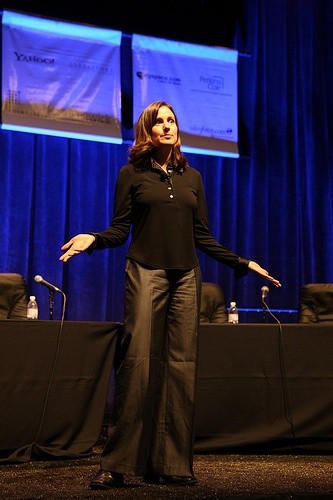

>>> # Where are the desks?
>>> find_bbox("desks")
[0,319,333,465]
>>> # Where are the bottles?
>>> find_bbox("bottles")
[27,295,38,320]
[228,302,239,324]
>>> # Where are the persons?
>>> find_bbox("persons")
[59,102,282,488]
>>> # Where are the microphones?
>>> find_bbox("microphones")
[261,285,269,302]
[34,275,62,293]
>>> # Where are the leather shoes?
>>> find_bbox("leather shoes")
[90,469,124,487]
[144,470,197,484]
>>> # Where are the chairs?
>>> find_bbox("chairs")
[0,272,27,319]
[198,281,228,323]
[297,283,333,323]
